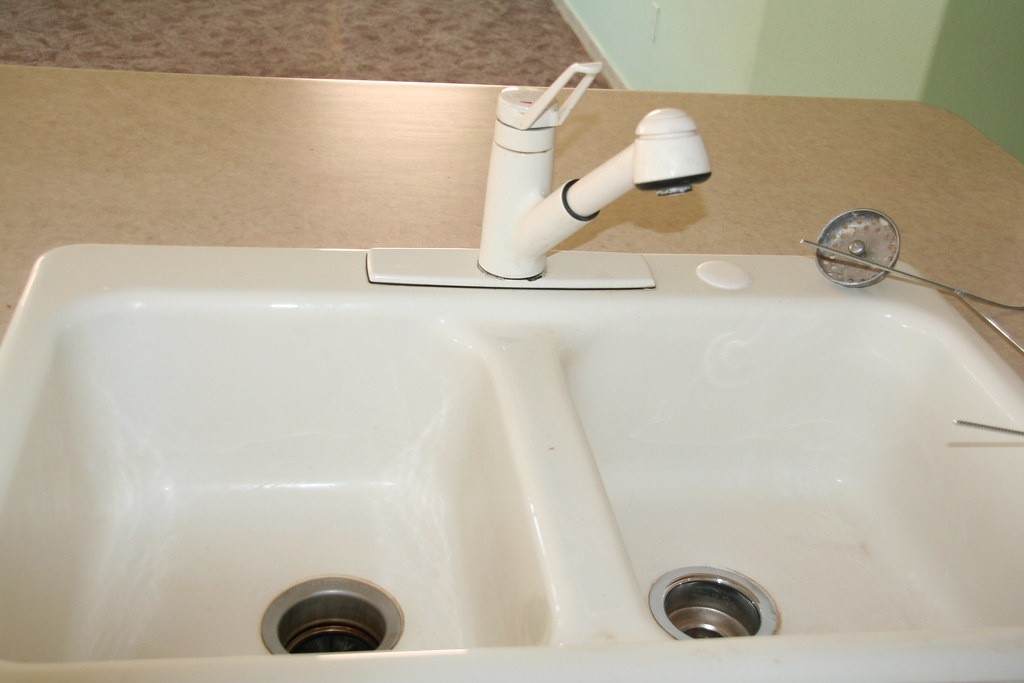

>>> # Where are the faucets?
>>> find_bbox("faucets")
[474,58,712,284]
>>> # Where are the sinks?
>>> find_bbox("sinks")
[1,236,596,682]
[548,247,1024,683]
